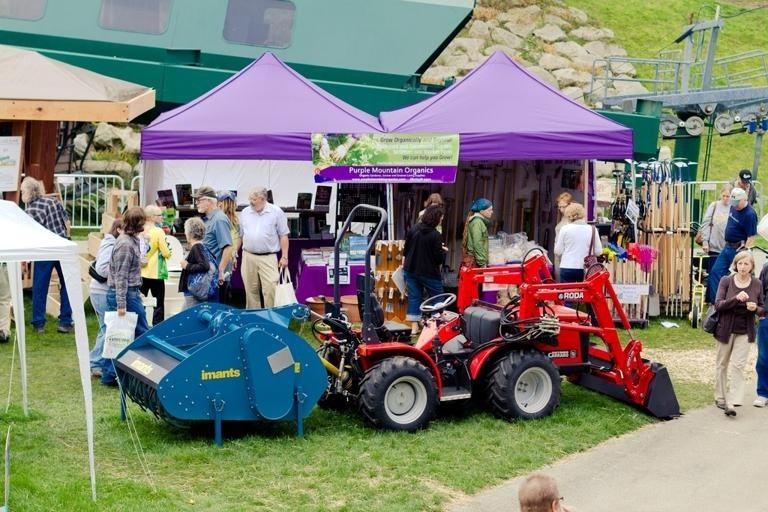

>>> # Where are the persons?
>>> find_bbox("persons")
[20,176,74,333]
[752,260,768,407]
[555,191,573,246]
[553,203,604,338]
[518,473,577,512]
[457,198,494,299]
[700,184,734,316]
[415,193,447,234]
[0,262,13,344]
[89,185,290,386]
[730,169,757,206]
[402,205,447,337]
[714,251,768,417]
[708,187,758,305]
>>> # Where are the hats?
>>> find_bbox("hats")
[190,185,217,198]
[730,188,746,206]
[739,170,752,182]
[471,199,491,212]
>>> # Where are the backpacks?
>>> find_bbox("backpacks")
[140,227,159,267]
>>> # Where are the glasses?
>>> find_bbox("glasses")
[195,199,208,204]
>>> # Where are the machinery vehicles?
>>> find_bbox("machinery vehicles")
[322,217,671,428]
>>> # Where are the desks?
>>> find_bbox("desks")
[294,250,366,305]
[224,205,336,291]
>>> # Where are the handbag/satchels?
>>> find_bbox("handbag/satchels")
[102,311,138,360]
[695,230,709,247]
[583,255,597,269]
[187,245,219,301]
[703,304,719,334]
[459,254,475,269]
[89,261,107,284]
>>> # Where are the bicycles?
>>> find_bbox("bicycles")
[688,248,711,328]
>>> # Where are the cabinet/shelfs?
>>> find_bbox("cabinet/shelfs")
[47,189,139,319]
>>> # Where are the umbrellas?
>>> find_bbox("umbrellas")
[756,212,768,243]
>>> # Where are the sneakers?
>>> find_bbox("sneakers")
[0,336,10,344]
[725,406,736,416]
[57,327,72,333]
[715,399,726,410]
[752,396,768,407]
[34,328,44,333]
[92,368,102,377]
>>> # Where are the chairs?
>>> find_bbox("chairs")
[355,274,412,343]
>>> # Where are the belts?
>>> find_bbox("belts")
[728,243,738,247]
[252,252,277,255]
